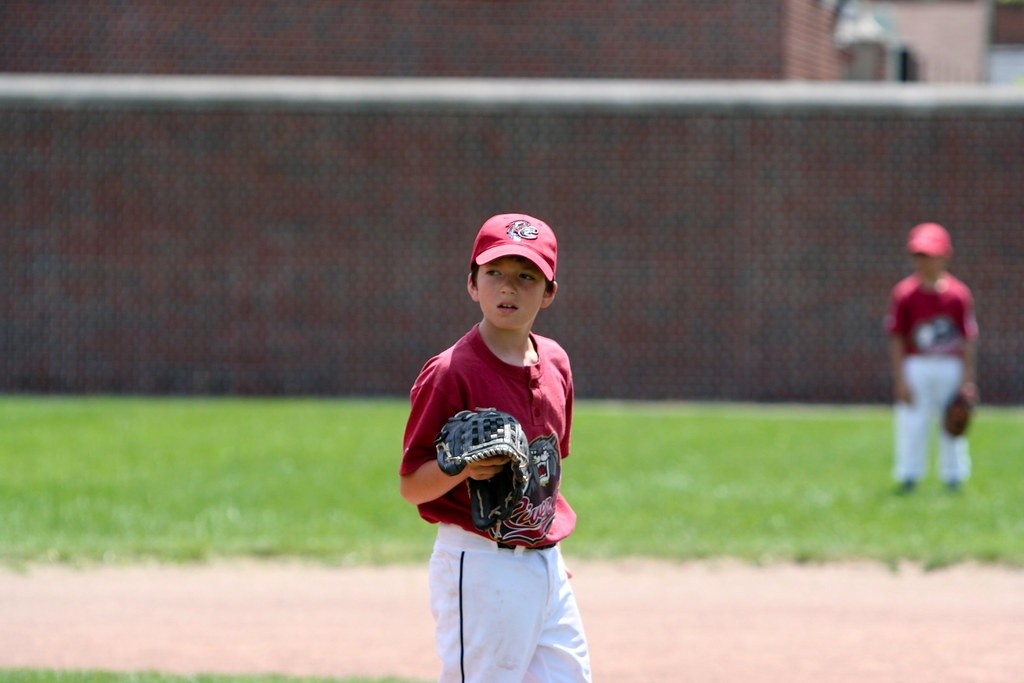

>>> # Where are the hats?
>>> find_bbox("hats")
[905,222,953,258]
[469,213,558,282]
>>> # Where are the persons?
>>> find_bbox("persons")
[400,214,592,683]
[885,222,979,495]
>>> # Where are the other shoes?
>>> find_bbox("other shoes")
[898,478,913,496]
[945,478,961,494]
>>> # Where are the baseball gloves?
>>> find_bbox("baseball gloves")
[433,405,532,533]
[944,393,971,437]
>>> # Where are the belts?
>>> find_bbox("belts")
[497,541,556,550]
